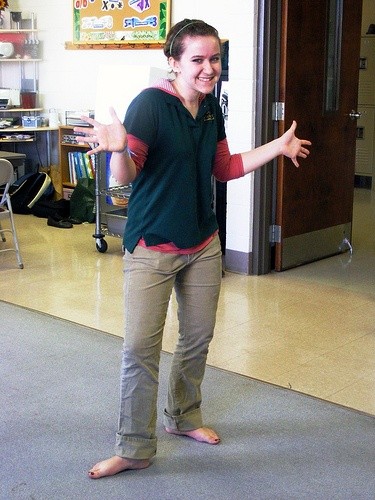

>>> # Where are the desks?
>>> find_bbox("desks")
[0,150,26,160]
[0,125,60,172]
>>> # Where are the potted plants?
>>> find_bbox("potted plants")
[19,91,37,109]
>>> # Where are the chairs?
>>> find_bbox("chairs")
[0,158,25,269]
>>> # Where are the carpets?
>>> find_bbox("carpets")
[1,300,375,500]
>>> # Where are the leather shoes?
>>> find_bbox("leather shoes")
[47,218,73,228]
[56,213,82,224]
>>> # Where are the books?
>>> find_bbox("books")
[68,151,96,186]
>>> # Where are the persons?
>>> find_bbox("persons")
[74,17,313,480]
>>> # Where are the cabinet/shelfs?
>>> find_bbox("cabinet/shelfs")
[0,12,46,114]
[58,124,96,201]
[353,4,375,192]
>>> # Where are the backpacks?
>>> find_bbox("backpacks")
[7,163,54,215]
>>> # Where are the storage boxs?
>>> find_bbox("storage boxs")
[63,188,73,200]
[22,115,43,128]
